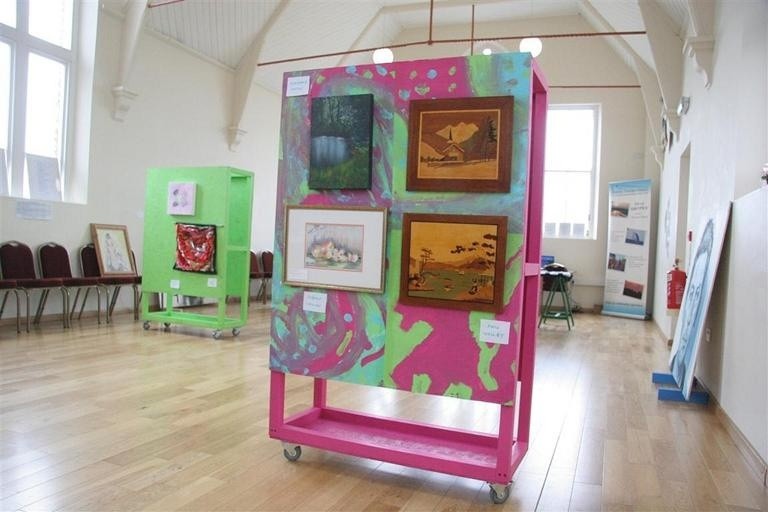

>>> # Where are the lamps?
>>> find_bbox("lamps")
[372,0,394,64]
[519,1,542,59]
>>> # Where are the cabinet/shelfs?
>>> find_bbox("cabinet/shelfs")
[143,165,253,330]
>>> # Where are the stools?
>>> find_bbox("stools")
[538,262,575,330]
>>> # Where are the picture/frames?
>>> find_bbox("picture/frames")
[405,95,514,194]
[281,205,387,294]
[90,223,138,278]
[399,211,508,314]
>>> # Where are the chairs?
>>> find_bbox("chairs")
[0,241,142,333]
[248,250,273,305]
